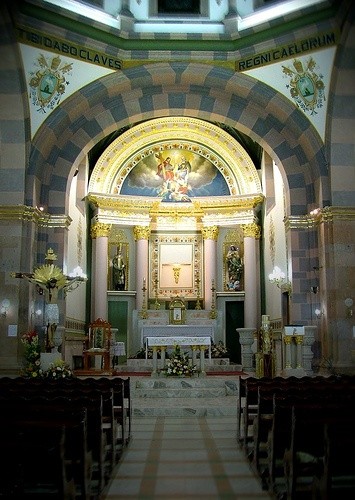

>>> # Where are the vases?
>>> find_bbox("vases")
[164,374,191,381]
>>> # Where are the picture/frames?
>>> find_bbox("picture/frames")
[148,232,204,302]
[221,241,245,293]
[107,241,130,291]
[172,306,182,322]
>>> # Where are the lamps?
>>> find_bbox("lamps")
[63,266,88,301]
[344,297,354,320]
[268,264,292,298]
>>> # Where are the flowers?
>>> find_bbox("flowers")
[18,328,43,380]
[157,344,200,378]
[128,342,154,359]
[41,359,79,380]
[196,337,232,358]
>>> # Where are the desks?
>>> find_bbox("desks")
[144,335,212,360]
[114,341,126,367]
[254,352,278,379]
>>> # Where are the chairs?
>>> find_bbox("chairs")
[81,317,113,371]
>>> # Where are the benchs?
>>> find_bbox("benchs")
[237,373,355,500]
[0,377,135,500]
